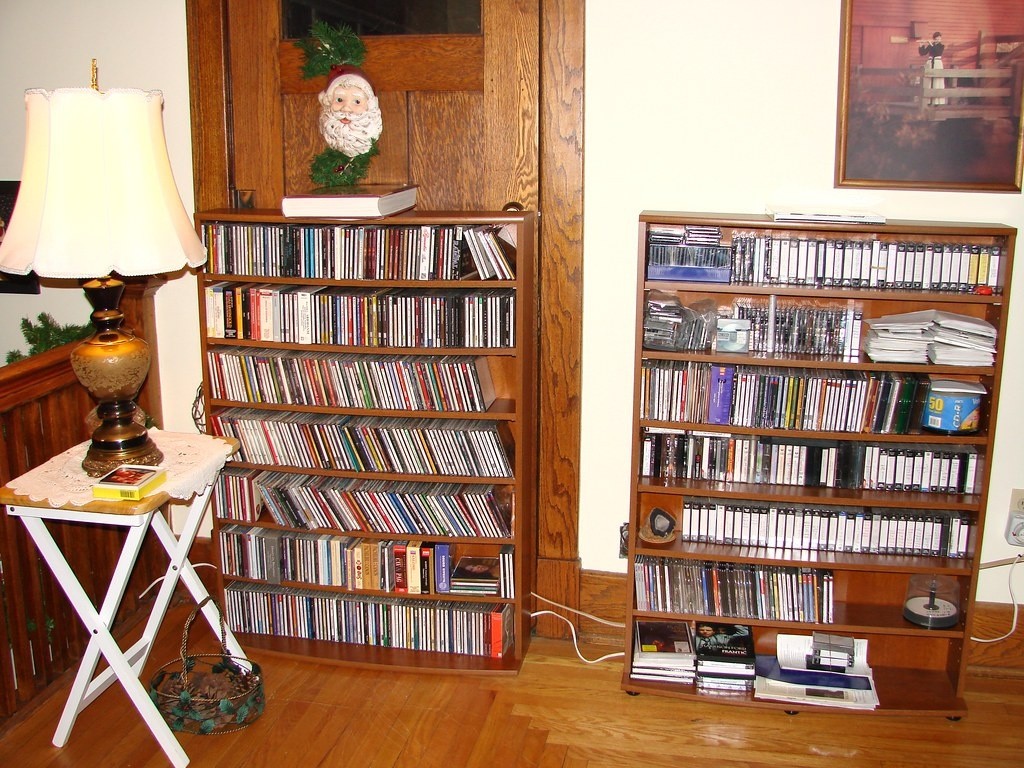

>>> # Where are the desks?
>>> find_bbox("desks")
[1,426,240,768]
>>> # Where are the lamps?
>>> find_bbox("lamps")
[0,59,208,477]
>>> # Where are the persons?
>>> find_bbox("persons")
[918,31,946,109]
[461,562,499,579]
[694,622,749,657]
[317,66,382,158]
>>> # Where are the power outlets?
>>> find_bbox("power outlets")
[1009,489,1024,512]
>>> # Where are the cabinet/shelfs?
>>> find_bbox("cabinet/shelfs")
[193,207,535,676]
[620,210,1018,721]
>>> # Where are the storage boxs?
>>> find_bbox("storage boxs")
[92,463,167,500]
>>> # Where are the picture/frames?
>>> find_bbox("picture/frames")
[834,0,1024,194]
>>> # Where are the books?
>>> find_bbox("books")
[629,223,1000,711]
[201,225,514,661]
[280,180,424,217]
